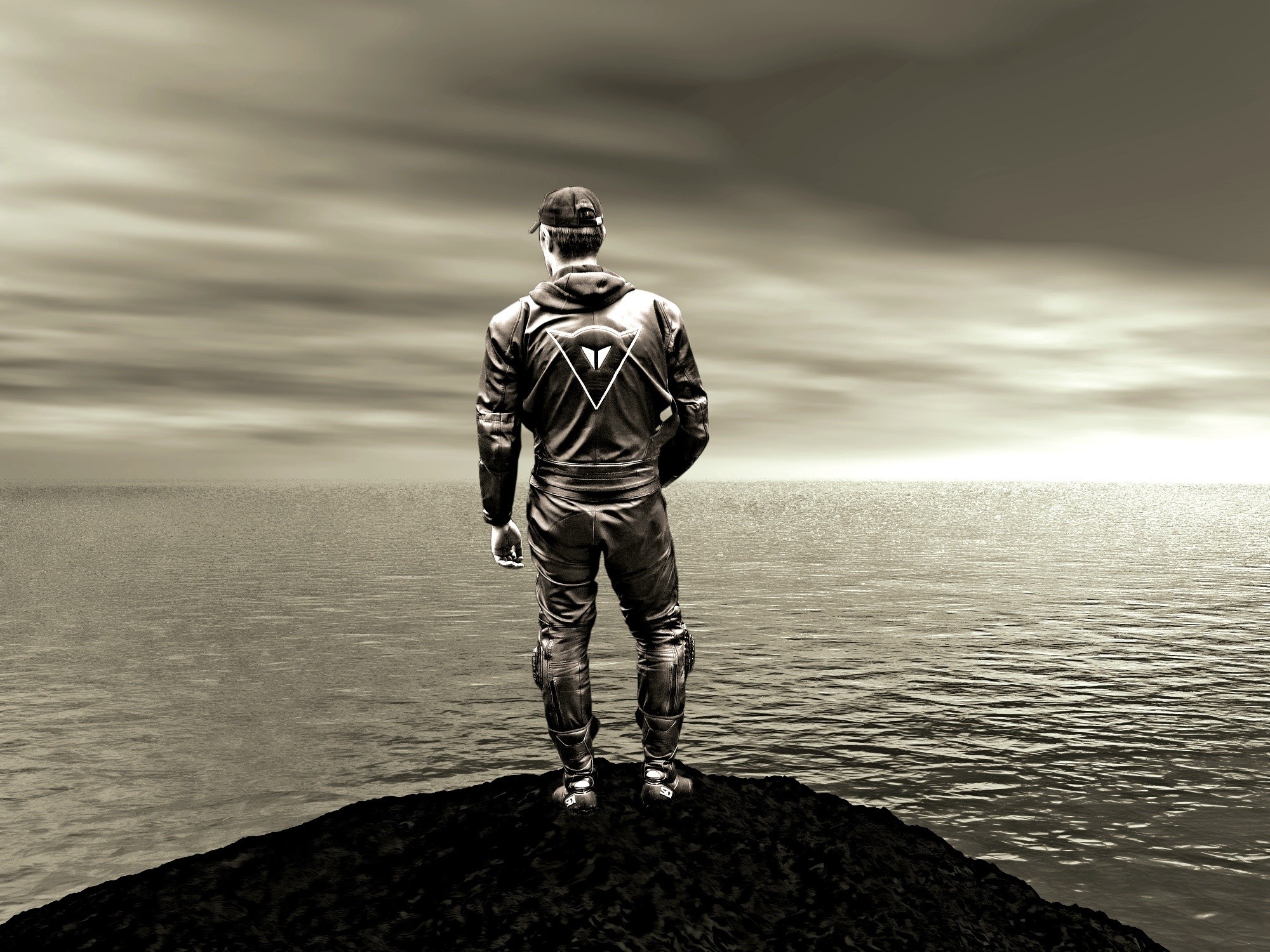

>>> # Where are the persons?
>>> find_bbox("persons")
[475,186,708,808]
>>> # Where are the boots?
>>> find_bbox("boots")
[634,706,695,808]
[547,715,601,819]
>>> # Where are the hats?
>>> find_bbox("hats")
[528,186,604,234]
